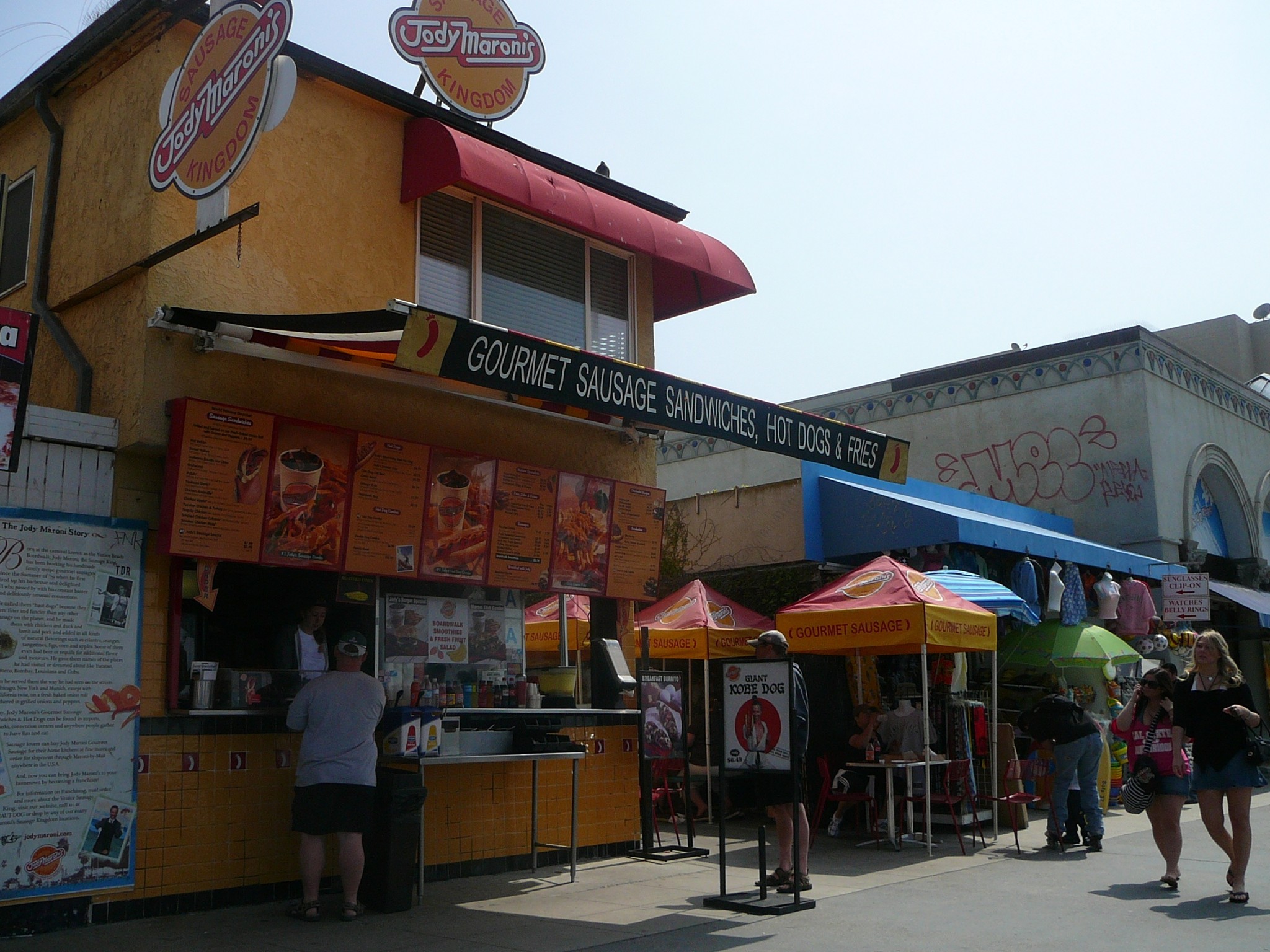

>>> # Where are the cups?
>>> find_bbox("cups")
[389,603,406,627]
[471,611,486,633]
[279,449,324,514]
[436,471,471,532]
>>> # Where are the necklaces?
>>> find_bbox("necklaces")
[1202,674,1216,681]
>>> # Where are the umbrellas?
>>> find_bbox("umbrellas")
[995,614,1143,677]
[919,566,1041,627]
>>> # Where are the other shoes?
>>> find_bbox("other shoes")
[725,810,740,819]
[1083,836,1090,845]
[1046,837,1059,849]
[1090,839,1103,850]
[1059,834,1080,844]
[693,809,714,822]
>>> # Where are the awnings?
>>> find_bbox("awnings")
[1208,577,1270,628]
[400,115,756,323]
[145,296,909,488]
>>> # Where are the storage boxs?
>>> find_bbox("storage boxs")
[379,703,443,758]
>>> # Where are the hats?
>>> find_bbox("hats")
[747,631,789,649]
[853,705,877,716]
[337,629,367,657]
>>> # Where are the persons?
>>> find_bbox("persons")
[823,703,905,837]
[878,700,934,780]
[747,630,812,893]
[881,544,1121,620]
[275,598,387,920]
[658,694,743,824]
[1018,629,1268,903]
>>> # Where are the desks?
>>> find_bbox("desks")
[846,758,952,850]
[380,751,585,906]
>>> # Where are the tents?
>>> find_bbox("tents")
[524,591,591,704]
[775,555,998,857]
[634,579,776,825]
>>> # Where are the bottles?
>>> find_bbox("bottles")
[873,737,881,764]
[486,680,493,708]
[420,675,431,707]
[439,682,446,708]
[500,677,508,708]
[866,740,875,763]
[515,674,527,708]
[446,682,463,708]
[876,715,888,722]
[464,681,478,708]
[431,678,439,708]
[478,680,486,708]
[410,675,420,707]
[526,676,541,708]
[494,677,500,709]
[508,678,516,708]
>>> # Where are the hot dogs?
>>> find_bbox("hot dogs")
[426,524,491,566]
[261,504,339,555]
[396,625,417,644]
[237,446,268,482]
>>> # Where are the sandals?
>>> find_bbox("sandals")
[288,901,323,921]
[777,869,812,893]
[338,902,365,920]
[755,867,796,887]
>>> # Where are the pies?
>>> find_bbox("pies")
[644,700,682,750]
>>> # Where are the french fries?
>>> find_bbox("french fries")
[556,505,605,573]
[461,469,492,517]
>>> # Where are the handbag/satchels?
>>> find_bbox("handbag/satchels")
[1119,777,1156,814]
[1239,716,1270,766]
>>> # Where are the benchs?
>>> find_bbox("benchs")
[659,775,746,783]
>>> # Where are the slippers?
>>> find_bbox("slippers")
[1229,891,1249,903]
[1226,868,1235,887]
[1161,875,1180,888]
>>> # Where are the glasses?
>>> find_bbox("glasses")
[1140,678,1161,689]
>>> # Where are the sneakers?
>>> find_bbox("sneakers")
[827,810,845,838]
[872,821,900,834]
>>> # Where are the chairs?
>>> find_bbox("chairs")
[898,758,987,856]
[809,756,885,854]
[971,759,1066,854]
[653,757,697,846]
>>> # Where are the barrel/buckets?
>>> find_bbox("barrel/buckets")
[1108,762,1123,808]
[1111,746,1129,765]
[1107,686,1121,697]
[1111,707,1124,717]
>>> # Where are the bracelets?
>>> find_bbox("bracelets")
[1244,709,1251,721]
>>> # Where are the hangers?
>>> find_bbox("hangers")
[950,690,986,710]
